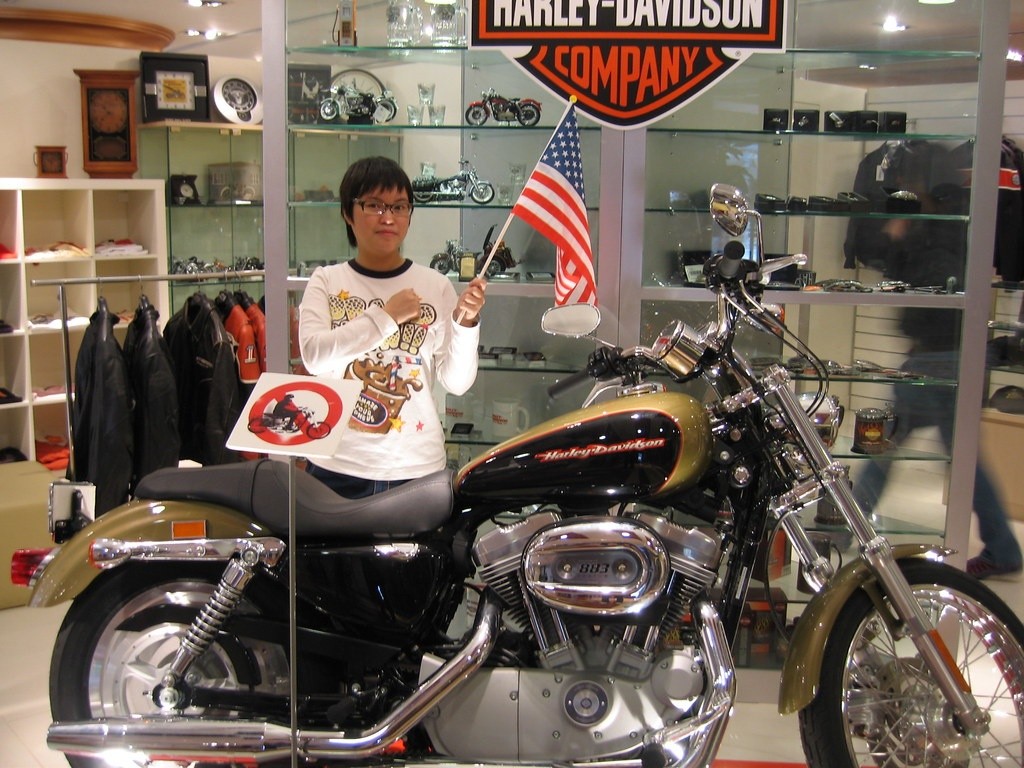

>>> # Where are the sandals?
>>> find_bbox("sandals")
[966,548,1022,580]
[826,530,854,553]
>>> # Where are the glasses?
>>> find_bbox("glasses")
[352,197,414,218]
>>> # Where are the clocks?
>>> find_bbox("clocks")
[139,51,212,123]
[72,68,141,179]
[33,145,70,179]
[331,69,387,125]
[166,172,201,205]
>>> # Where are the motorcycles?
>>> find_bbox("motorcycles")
[10,181,1024,768]
[409,156,496,204]
[429,223,519,280]
[169,248,266,284]
[465,87,544,127]
[318,77,397,123]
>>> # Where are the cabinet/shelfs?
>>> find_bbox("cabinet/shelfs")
[0,177,170,479]
[620,0,1012,706]
[137,121,405,326]
[260,0,624,520]
[942,326,1024,521]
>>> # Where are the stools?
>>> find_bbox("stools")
[0,460,60,610]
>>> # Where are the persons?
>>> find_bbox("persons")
[298,156,488,500]
[848,141,1024,580]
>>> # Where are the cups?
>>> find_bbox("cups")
[428,105,446,125]
[850,407,899,456]
[385,0,424,47]
[492,397,530,437]
[796,533,842,595]
[430,3,468,47]
[816,464,853,526]
[509,163,526,184]
[418,84,435,105]
[408,105,424,125]
[445,392,484,428]
[495,184,514,204]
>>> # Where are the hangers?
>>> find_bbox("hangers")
[188,271,208,319]
[213,268,235,317]
[232,271,249,305]
[96,273,109,312]
[137,273,149,309]
[885,135,1016,159]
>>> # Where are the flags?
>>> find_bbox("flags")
[509,103,601,314]
